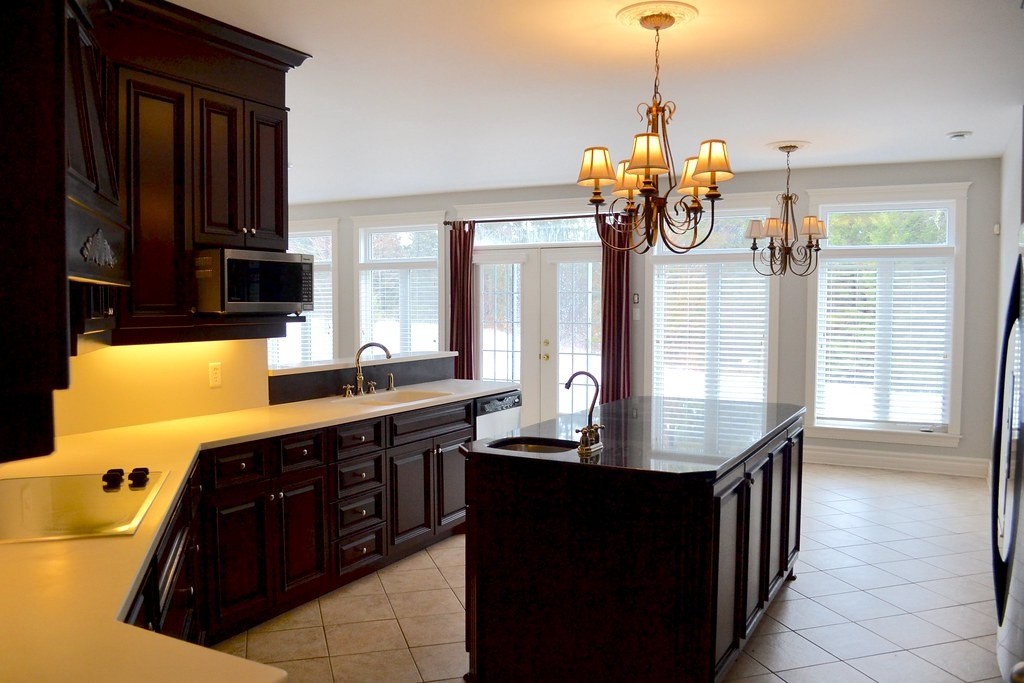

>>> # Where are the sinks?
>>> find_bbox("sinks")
[489,436,582,454]
[331,389,452,406]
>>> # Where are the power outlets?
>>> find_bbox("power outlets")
[209,362,222,387]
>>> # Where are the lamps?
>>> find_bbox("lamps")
[744,140,829,277]
[576,1,736,255]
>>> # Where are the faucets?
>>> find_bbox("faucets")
[355,342,393,396]
[565,371,600,430]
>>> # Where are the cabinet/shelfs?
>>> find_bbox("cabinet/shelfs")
[0,373,523,683]
[88,0,315,346]
[455,394,808,683]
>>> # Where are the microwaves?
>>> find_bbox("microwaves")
[191,248,314,316]
